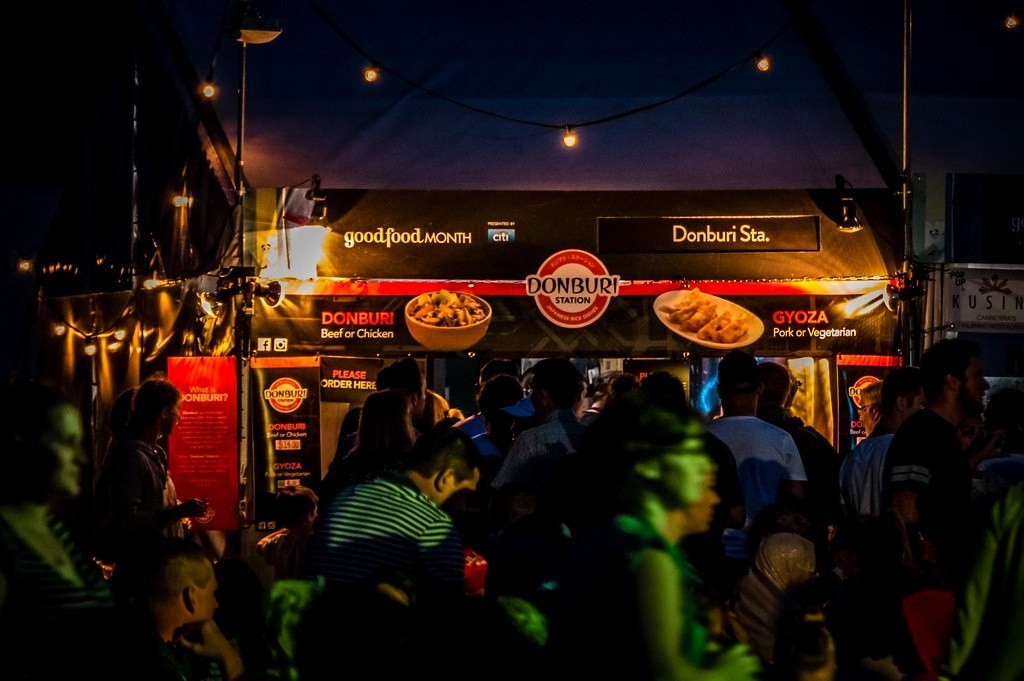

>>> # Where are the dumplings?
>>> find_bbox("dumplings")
[658,287,748,343]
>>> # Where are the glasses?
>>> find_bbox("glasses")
[526,384,535,394]
[474,383,482,394]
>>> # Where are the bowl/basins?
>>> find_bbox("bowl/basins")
[405,291,493,348]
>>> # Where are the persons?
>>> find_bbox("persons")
[309,427,482,605]
[77,534,248,681]
[547,371,837,681]
[334,393,416,487]
[838,365,926,516]
[0,363,113,608]
[491,358,588,490]
[107,372,207,562]
[376,357,427,445]
[756,360,843,519]
[470,375,523,455]
[885,339,1024,681]
[452,357,520,437]
[256,486,319,567]
[707,350,808,561]
[323,409,362,488]
[860,383,883,438]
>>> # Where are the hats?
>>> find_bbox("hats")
[718,350,761,393]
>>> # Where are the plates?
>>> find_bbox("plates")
[653,290,764,348]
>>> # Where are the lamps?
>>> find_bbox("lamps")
[200,286,240,318]
[252,281,285,307]
[289,174,328,219]
[835,174,864,234]
[882,283,923,311]
